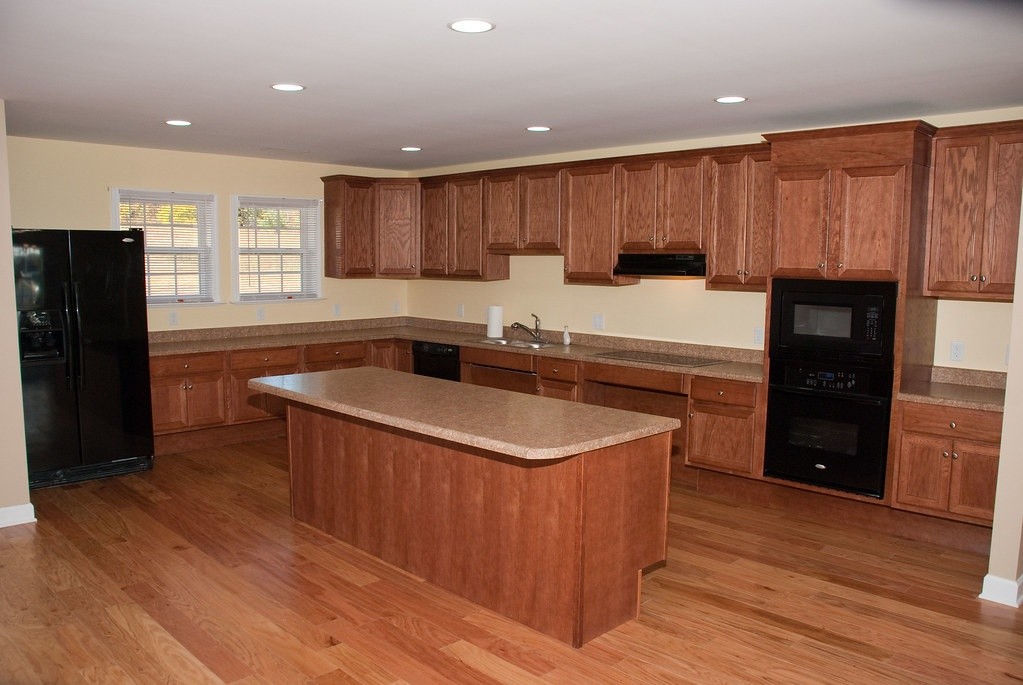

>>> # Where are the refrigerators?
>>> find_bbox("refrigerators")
[13,228,154,489]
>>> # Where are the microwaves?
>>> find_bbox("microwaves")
[767,278,899,364]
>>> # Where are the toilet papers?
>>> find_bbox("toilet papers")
[486,305,503,338]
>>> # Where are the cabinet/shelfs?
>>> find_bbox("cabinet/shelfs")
[369,338,394,370]
[762,120,938,281]
[394,338,413,374]
[618,148,705,254]
[892,400,1004,528]
[923,118,1023,303]
[705,142,772,292]
[320,175,377,280]
[227,346,302,425]
[302,341,369,372]
[377,177,419,280]
[419,170,510,282]
[149,351,228,436]
[685,373,764,479]
[537,355,581,402]
[564,157,640,286]
[486,162,564,255]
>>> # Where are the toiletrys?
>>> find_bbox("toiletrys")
[562,325,571,345]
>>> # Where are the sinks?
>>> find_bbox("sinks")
[470,338,557,349]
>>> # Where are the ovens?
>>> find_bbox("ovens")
[763,360,899,501]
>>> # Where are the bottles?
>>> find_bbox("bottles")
[562,326,571,345]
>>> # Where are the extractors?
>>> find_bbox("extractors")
[613,252,706,280]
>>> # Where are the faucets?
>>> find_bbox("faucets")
[511,312,550,344]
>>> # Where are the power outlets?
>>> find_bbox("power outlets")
[951,342,964,361]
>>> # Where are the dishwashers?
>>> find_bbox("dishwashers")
[411,341,460,382]
